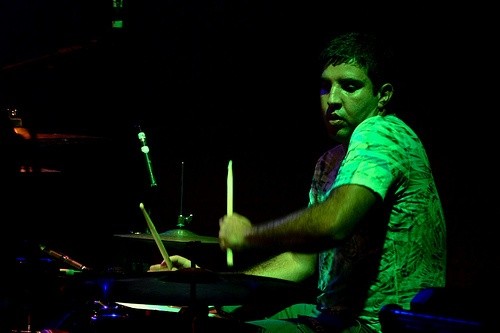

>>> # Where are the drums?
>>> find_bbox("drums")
[113,302,222,333]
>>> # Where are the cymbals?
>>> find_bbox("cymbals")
[82,271,323,308]
[113,228,222,252]
[0,126,105,145]
[17,165,62,174]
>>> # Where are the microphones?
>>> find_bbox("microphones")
[138,132,157,187]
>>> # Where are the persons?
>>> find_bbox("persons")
[161,32,447,333]
